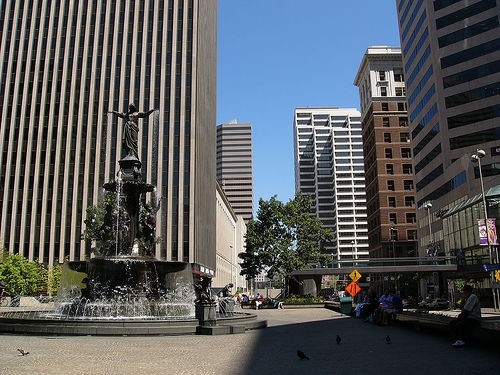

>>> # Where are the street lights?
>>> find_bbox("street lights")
[390,227,398,295]
[470,149,497,312]
[330,253,334,268]
[424,203,433,244]
[351,240,355,266]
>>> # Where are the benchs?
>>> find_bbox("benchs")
[395,312,457,336]
[324,301,341,312]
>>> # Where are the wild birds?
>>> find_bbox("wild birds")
[297,349,310,360]
[336,334,341,343]
[16,348,28,356]
[385,336,391,344]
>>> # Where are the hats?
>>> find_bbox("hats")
[461,285,473,292]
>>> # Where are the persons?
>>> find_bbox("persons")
[354,286,402,326]
[108,103,156,159]
[139,200,161,241]
[270,298,283,309]
[222,282,233,297]
[448,285,481,346]
[241,294,249,309]
[194,273,213,301]
[237,296,240,302]
[251,293,265,310]
[99,204,131,251]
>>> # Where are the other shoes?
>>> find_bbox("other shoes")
[451,340,464,345]
[384,322,388,324]
